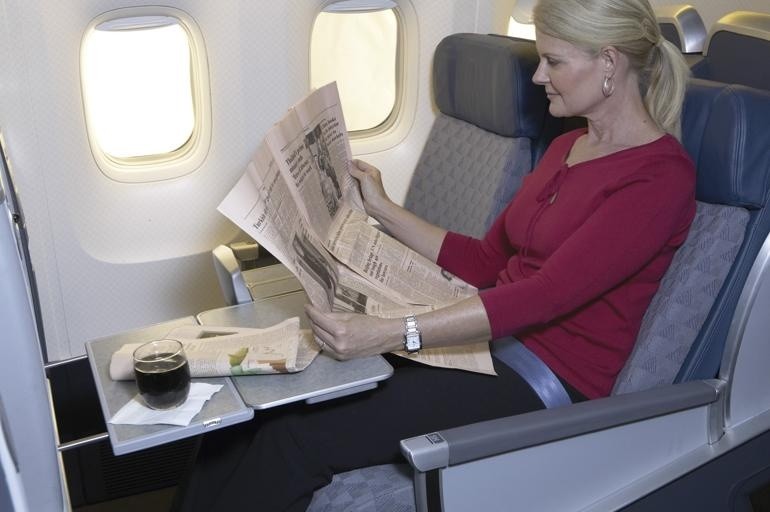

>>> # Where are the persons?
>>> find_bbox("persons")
[168,0,698,512]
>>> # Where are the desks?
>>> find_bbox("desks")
[43,293,397,459]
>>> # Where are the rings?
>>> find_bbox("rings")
[318,342,327,350]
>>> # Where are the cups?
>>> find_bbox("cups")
[133,338,192,412]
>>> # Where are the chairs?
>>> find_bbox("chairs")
[212,4,770,512]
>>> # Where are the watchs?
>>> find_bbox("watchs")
[402,314,423,356]
